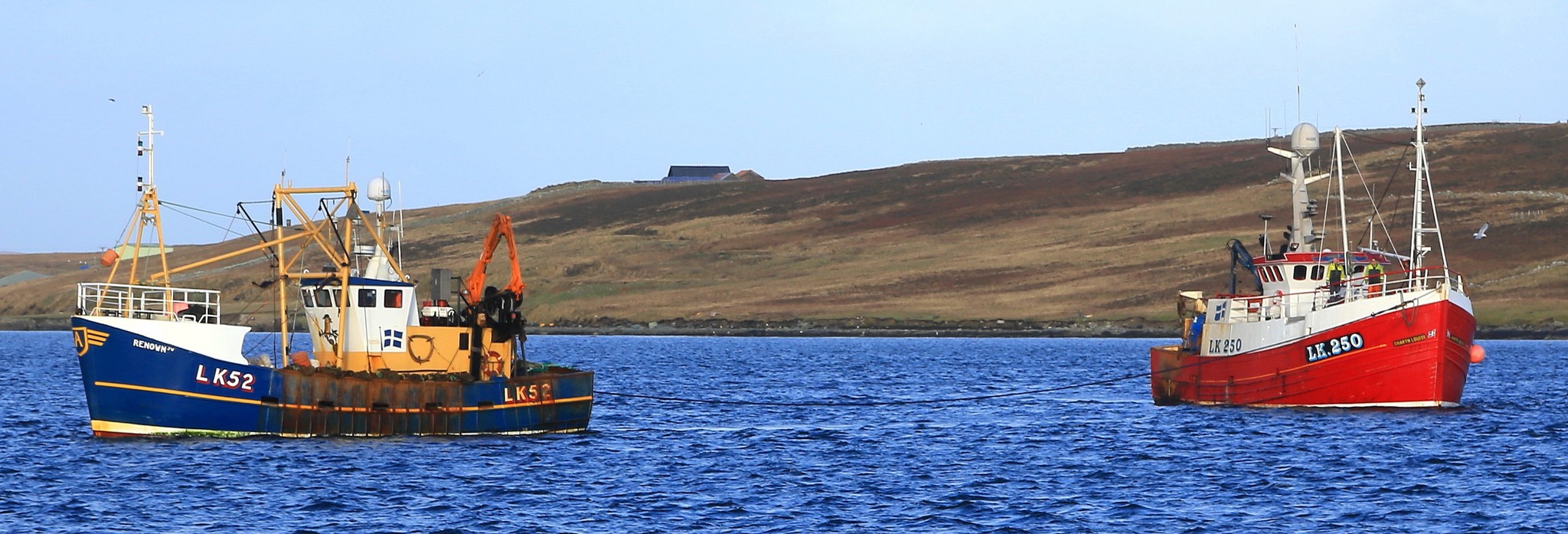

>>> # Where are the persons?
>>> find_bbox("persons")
[1325,258,1346,303]
[1364,258,1384,299]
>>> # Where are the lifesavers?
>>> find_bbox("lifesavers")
[1272,290,1283,304]
[483,351,503,377]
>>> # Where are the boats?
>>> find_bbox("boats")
[70,103,595,443]
[1151,77,1491,412]
